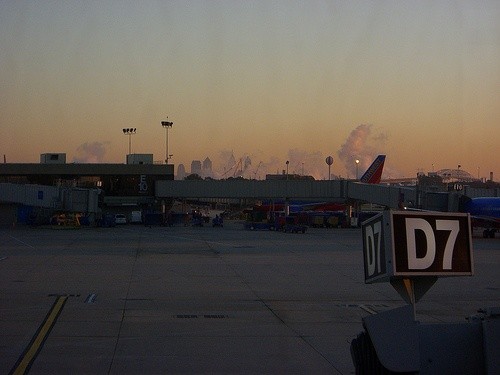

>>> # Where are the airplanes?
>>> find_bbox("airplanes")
[232,153,387,224]
[465,196,500,238]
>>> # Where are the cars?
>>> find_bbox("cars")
[32,202,358,234]
